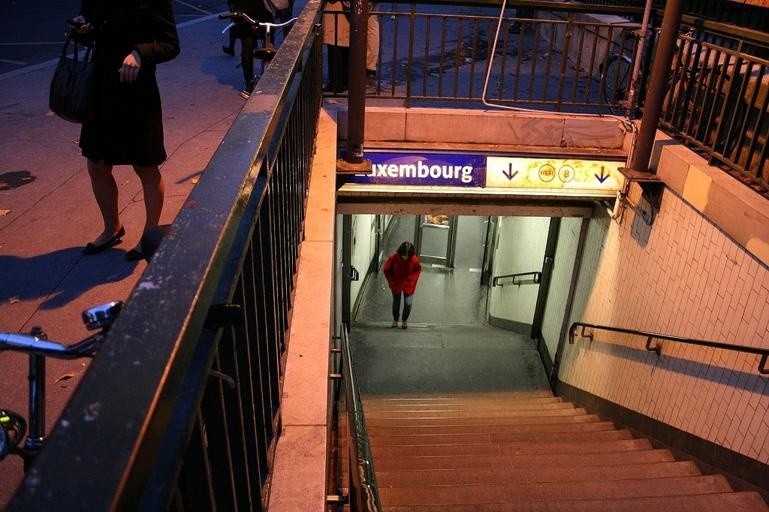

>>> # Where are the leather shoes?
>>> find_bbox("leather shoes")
[124,241,157,262]
[85,225,125,255]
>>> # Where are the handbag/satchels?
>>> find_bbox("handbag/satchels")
[48,57,98,125]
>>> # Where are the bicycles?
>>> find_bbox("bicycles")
[0,223,242,479]
[218,12,322,88]
[598,26,746,155]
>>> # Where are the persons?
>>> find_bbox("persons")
[0,406,29,464]
[259,0,296,58]
[384,242,422,330]
[69,0,183,263]
[220,1,259,100]
[320,0,379,93]
[506,8,535,35]
